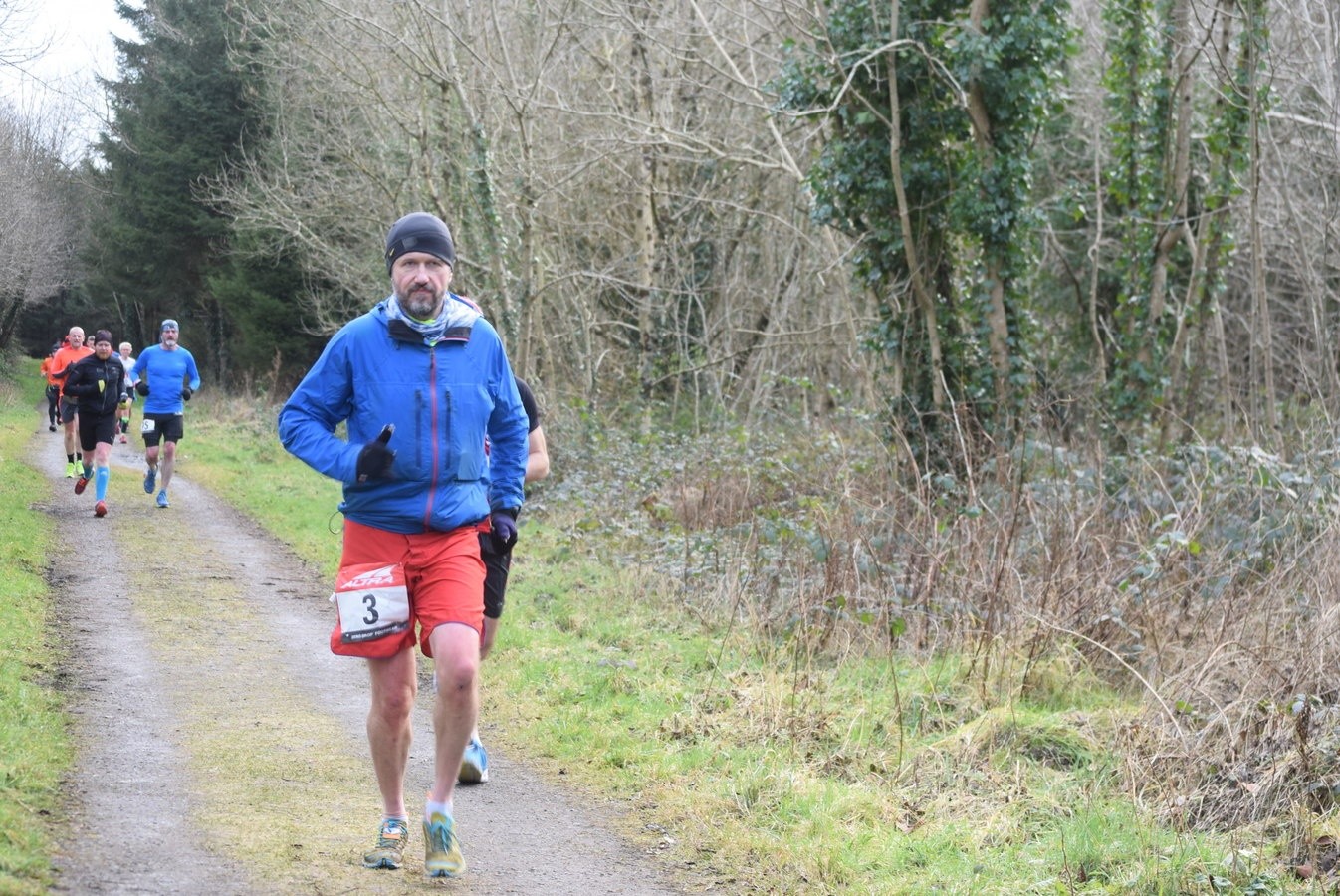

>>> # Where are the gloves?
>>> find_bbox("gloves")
[94,380,105,394]
[67,362,74,371]
[358,424,400,481]
[136,382,150,397]
[181,388,191,401]
[118,397,128,410]
[491,509,519,555]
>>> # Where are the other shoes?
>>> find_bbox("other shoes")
[120,436,128,443]
[116,421,121,434]
[122,421,129,432]
[50,425,56,431]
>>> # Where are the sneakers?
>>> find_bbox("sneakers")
[423,812,465,879]
[95,501,107,517]
[459,742,489,783]
[75,462,85,476]
[157,490,170,508]
[144,463,158,493]
[74,470,94,495]
[64,461,74,477]
[362,818,408,869]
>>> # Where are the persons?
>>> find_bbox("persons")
[277,213,531,882]
[453,296,551,788]
[40,326,96,478]
[110,341,137,444]
[62,330,129,518]
[129,318,202,510]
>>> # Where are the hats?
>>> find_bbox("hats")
[94,330,111,345]
[161,319,180,332]
[385,212,454,270]
